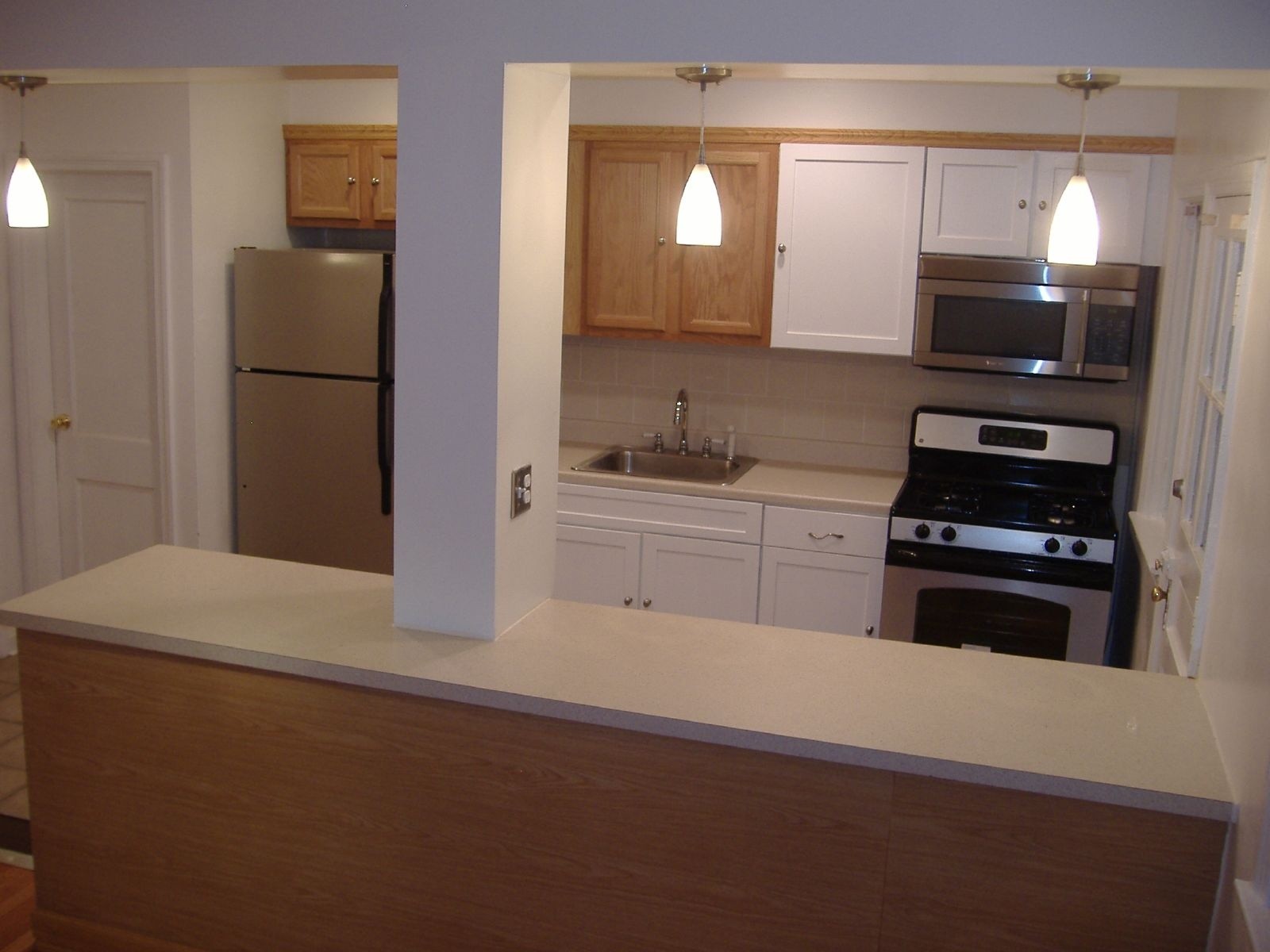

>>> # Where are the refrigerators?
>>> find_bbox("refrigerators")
[233,246,394,576]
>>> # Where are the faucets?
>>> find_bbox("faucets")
[726,425,739,460]
[672,388,689,456]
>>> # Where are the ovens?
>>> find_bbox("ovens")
[879,541,1119,667]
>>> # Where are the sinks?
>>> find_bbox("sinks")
[569,445,760,486]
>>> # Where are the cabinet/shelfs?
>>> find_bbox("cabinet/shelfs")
[764,124,927,362]
[921,128,1152,269]
[756,502,891,641]
[544,483,762,637]
[280,121,397,233]
[583,121,769,353]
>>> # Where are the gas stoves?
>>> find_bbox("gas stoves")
[887,405,1121,565]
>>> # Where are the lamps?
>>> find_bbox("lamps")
[1045,70,1122,270]
[666,67,737,249]
[0,73,53,230]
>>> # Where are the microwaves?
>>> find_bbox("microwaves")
[912,254,1142,384]
